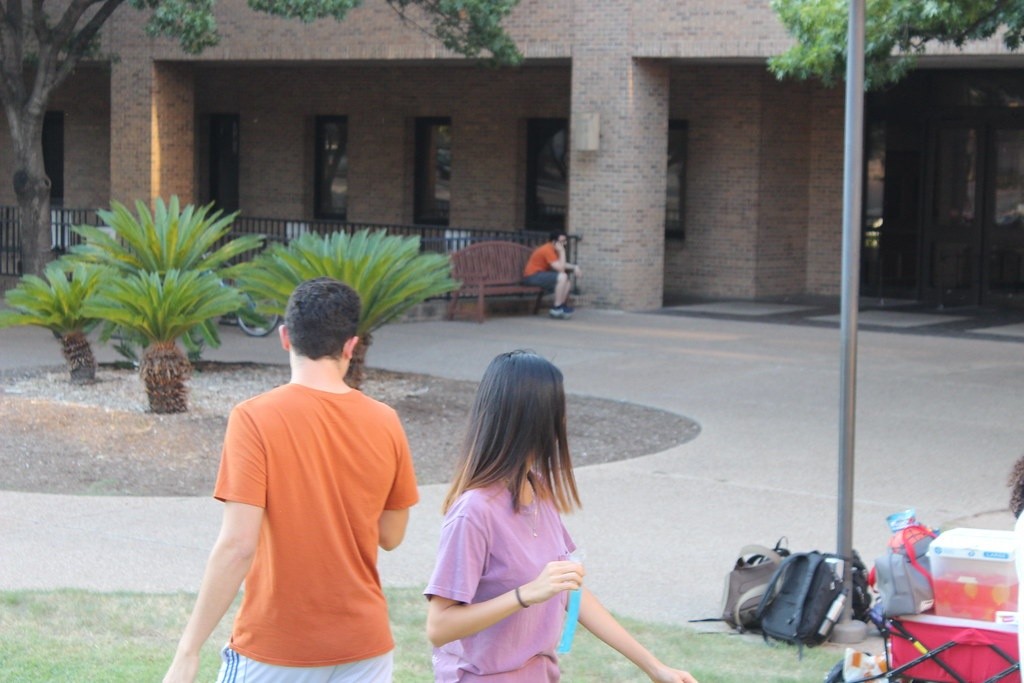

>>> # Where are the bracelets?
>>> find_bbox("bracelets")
[515,586,530,608]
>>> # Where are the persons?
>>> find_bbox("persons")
[522,230,581,319]
[427,350,698,683]
[1006,457,1024,683]
[160,278,418,683]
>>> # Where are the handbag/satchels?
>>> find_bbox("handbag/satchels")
[869,522,941,618]
[822,648,888,683]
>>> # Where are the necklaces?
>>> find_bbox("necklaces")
[505,477,538,538]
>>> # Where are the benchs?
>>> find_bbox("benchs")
[444,241,543,323]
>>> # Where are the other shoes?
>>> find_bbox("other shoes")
[550,305,573,319]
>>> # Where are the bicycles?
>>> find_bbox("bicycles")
[201,252,281,339]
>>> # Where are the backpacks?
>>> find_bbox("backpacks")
[722,536,875,661]
